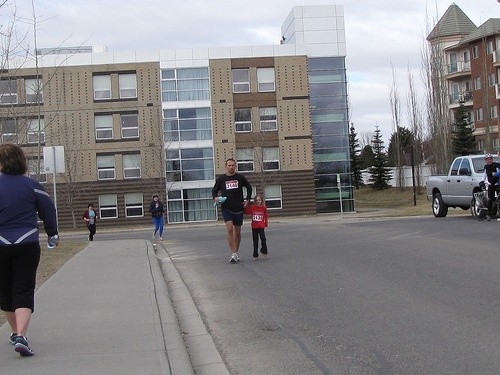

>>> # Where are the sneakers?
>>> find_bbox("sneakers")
[9,331,17,344]
[15,335,34,356]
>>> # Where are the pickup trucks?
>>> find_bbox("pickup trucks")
[426,154,500,218]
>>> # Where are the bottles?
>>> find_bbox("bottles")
[47,234,58,249]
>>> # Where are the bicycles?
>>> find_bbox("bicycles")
[470,183,500,223]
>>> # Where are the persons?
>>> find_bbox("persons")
[483,153,500,221]
[148,195,165,246]
[243,195,268,258]
[0,144,61,356]
[83,203,98,241]
[212,157,253,264]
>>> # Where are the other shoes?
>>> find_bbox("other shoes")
[487,216,491,221]
[236,252,240,260]
[230,253,238,263]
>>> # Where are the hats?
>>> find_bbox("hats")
[484,154,493,160]
